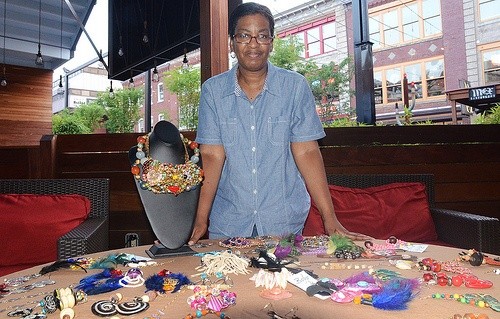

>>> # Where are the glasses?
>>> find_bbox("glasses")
[232,33,275,45]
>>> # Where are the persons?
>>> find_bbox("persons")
[187,2,375,245]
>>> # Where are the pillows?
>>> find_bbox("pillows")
[0,193,90,267]
[302,182,437,242]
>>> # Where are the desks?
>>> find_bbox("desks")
[0,235,499,319]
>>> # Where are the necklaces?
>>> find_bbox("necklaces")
[130,131,205,196]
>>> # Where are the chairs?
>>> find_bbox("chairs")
[327,174,498,254]
[0,177,109,278]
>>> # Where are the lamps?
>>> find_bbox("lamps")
[109,21,190,99]
[0,0,7,87]
[58,0,65,96]
[35,0,43,65]
